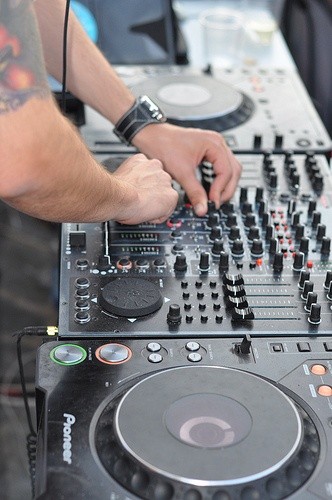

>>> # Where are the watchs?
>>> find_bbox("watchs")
[111,93,168,147]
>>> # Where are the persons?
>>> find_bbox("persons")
[0,0,242,499]
